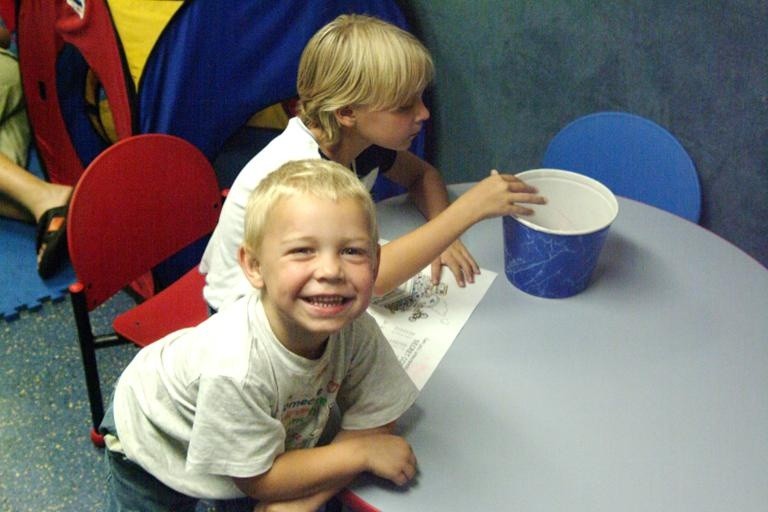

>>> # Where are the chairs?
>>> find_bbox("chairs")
[542,112,702,225]
[67,134,231,447]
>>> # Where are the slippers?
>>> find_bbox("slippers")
[34,202,75,275]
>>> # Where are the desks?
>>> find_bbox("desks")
[321,182,767,510]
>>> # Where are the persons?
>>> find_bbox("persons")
[1,18,74,281]
[198,14,548,319]
[98,160,421,512]
